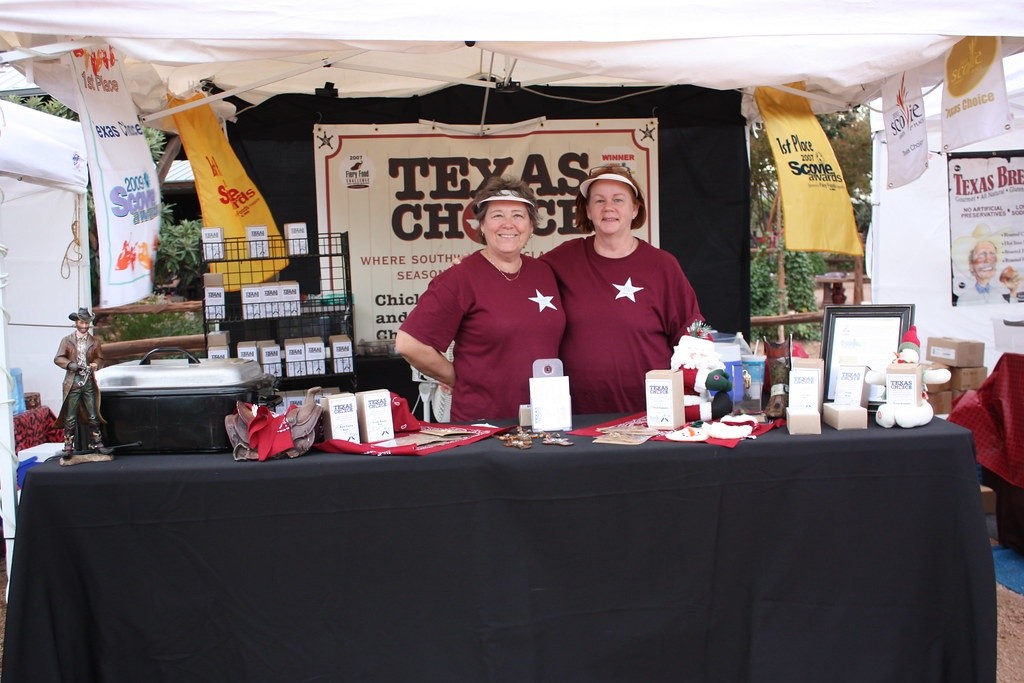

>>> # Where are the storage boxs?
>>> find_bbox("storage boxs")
[200,226,394,445]
[646,333,987,430]
[518,375,573,432]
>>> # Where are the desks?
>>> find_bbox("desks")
[0,414,998,683]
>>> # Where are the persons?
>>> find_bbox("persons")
[50,306,116,461]
[395,172,567,424]
[537,166,707,414]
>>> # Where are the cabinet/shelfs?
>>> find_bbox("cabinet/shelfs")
[199,231,356,381]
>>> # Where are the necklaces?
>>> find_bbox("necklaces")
[593,236,635,261]
[485,247,521,282]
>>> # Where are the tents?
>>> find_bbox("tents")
[1,90,95,580]
[0,1,1023,453]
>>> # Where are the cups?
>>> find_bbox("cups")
[740,355,766,399]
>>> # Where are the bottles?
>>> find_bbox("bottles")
[732,332,753,356]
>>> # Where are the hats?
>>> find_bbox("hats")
[476,188,535,209]
[580,172,638,197]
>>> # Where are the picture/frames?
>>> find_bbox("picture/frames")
[820,304,916,414]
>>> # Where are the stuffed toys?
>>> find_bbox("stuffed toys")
[670,327,732,422]
[862,325,953,429]
